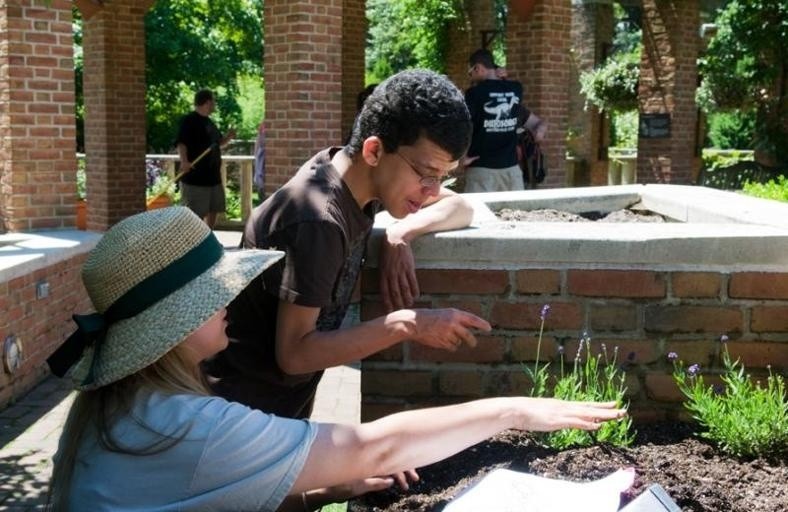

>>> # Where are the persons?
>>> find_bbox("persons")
[171,86,237,233]
[42,203,628,511]
[354,83,379,116]
[253,117,267,204]
[492,62,551,190]
[208,65,492,425]
[464,46,524,193]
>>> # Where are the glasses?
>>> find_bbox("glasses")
[392,149,457,188]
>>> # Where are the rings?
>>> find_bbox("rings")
[455,337,462,345]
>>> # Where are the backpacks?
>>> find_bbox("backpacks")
[523,141,546,185]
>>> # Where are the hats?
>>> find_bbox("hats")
[46,206,289,393]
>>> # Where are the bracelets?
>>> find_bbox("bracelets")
[301,490,322,511]
